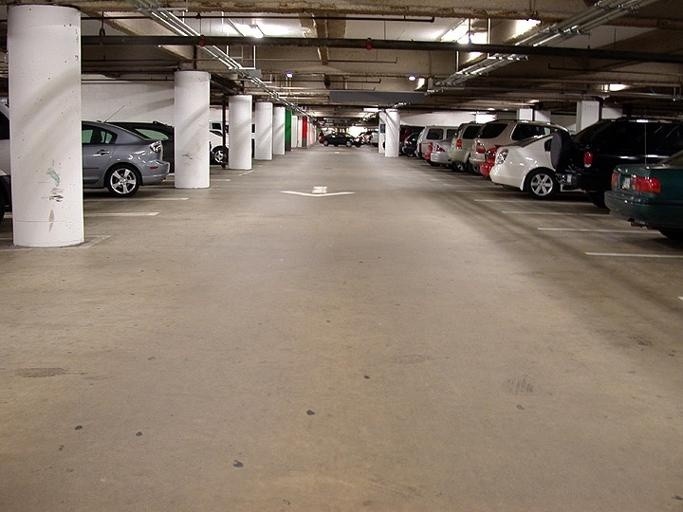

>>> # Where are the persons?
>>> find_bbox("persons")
[317,129,324,138]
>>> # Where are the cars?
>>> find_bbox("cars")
[319,131,363,148]
[359,129,378,148]
[209,128,229,167]
[81,120,174,198]
[0,101,11,222]
[400,118,682,239]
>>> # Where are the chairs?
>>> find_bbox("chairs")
[90,128,101,144]
